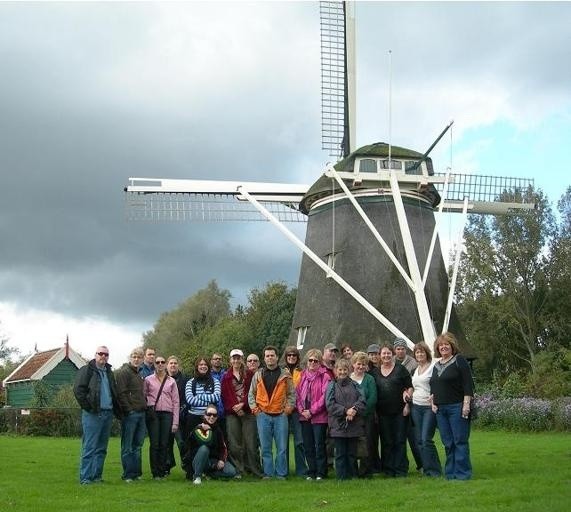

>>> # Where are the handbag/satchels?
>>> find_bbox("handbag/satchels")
[180,404,188,424]
[146,405,155,423]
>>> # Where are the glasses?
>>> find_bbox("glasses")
[309,359,318,363]
[206,413,217,417]
[287,354,297,356]
[247,360,258,362]
[155,361,165,364]
[98,352,109,357]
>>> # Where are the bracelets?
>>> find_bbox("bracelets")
[463,400,470,406]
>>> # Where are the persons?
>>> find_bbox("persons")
[402,342,442,476]
[114,349,147,483]
[137,338,422,486]
[73,347,118,485]
[428,332,474,481]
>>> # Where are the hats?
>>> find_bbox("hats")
[230,349,243,356]
[367,343,380,354]
[393,337,407,349]
[325,342,339,352]
